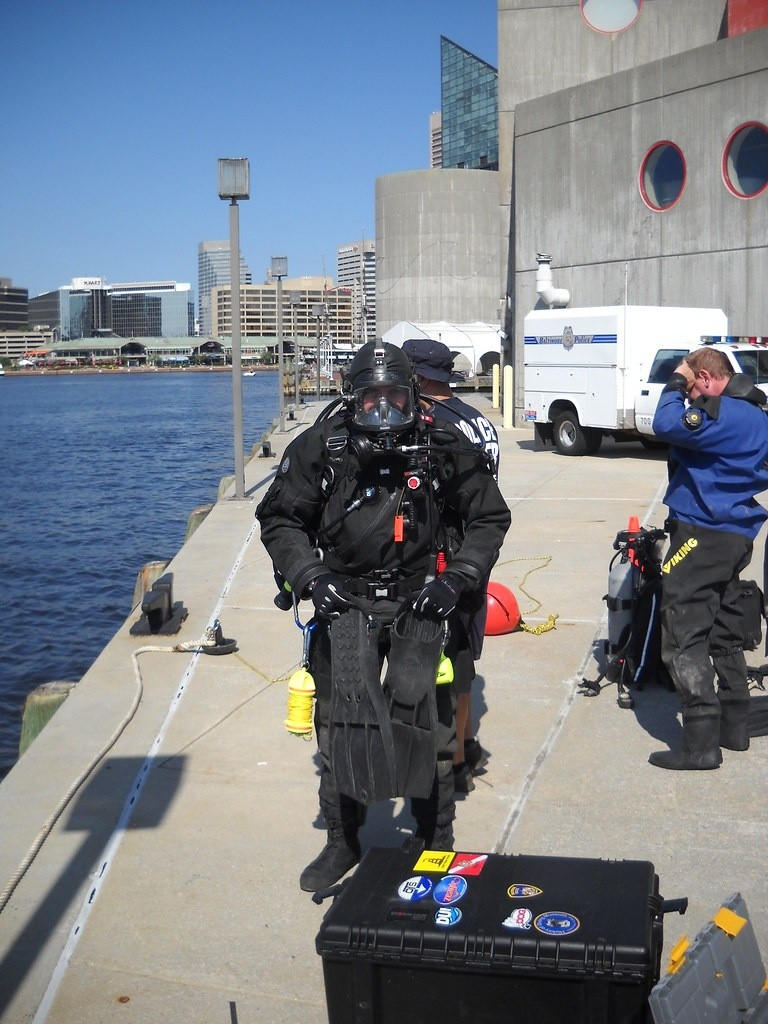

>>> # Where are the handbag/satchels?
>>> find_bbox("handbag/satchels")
[736,580,762,651]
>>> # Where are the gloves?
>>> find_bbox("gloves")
[411,573,464,620]
[311,577,349,618]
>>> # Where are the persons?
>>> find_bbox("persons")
[649,347,768,770]
[255,337,511,892]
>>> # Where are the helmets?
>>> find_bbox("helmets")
[350,337,418,433]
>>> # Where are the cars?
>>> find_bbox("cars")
[20,359,122,369]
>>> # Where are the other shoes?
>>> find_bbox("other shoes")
[301,826,362,892]
[718,724,750,750]
[645,748,722,768]
[423,824,455,852]
[465,740,488,770]
[449,762,474,793]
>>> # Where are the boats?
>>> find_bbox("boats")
[0,371,5,376]
[243,372,256,377]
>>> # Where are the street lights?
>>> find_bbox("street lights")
[270,255,290,435]
[286,291,304,411]
[312,304,325,402]
[216,157,256,501]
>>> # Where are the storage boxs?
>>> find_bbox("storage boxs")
[314,846,688,1024]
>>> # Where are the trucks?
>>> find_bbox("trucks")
[522,306,768,458]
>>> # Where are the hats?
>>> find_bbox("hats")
[400,339,455,382]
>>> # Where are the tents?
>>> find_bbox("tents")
[381,320,501,390]
